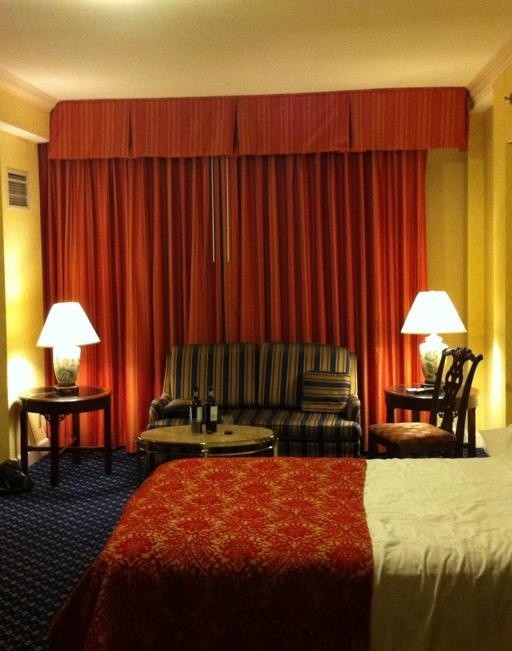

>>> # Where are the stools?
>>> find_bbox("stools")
[368,422,459,458]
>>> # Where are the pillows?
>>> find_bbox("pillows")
[300,370,351,414]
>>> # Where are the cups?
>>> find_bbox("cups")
[221,414,231,432]
[224,417,233,433]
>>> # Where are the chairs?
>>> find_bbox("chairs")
[395,347,484,458]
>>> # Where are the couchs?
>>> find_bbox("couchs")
[146,343,362,477]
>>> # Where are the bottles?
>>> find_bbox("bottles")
[204,388,219,433]
[189,387,203,433]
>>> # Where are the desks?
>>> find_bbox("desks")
[383,383,477,458]
[21,388,113,487]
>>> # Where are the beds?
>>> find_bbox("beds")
[45,425,512,651]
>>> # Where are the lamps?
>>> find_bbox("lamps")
[401,290,468,388]
[36,302,102,396]
